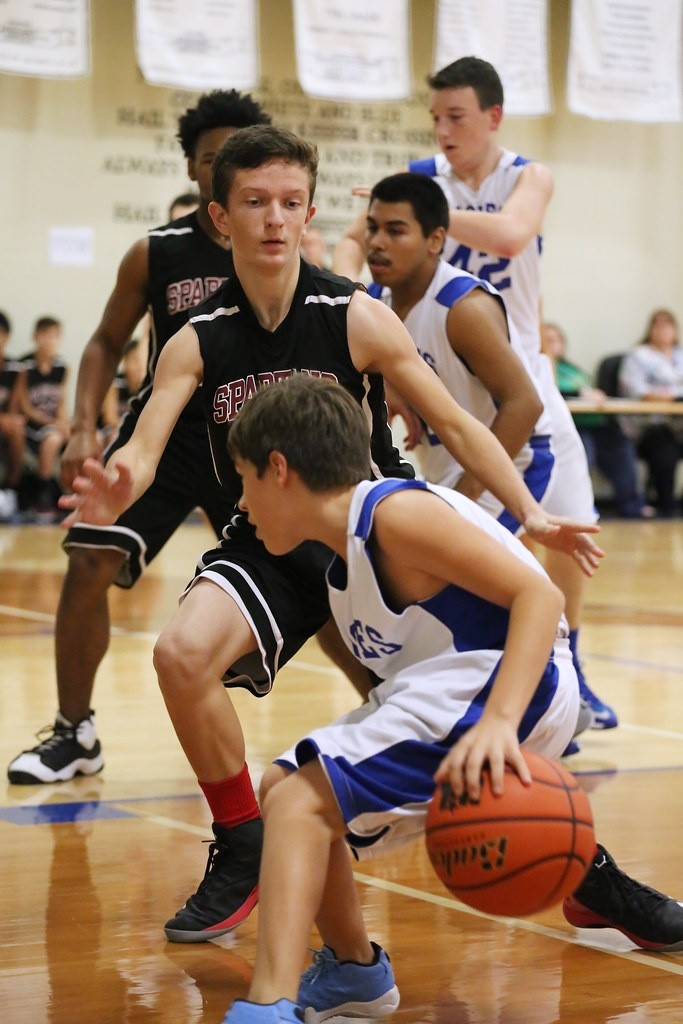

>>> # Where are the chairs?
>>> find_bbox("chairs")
[596,354,653,506]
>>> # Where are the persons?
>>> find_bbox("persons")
[57,125,683,951]
[538,325,657,520]
[6,90,424,785]
[364,172,558,541]
[0,312,148,524]
[618,311,683,519]
[332,56,617,731]
[215,379,580,1024]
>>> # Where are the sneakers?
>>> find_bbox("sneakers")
[562,842,683,951]
[222,997,304,1024]
[576,671,619,730]
[297,940,401,1024]
[7,709,105,784]
[165,818,265,942]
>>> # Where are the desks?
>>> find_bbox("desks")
[568,402,683,416]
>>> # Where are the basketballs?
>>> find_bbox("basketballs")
[425,744,594,916]
